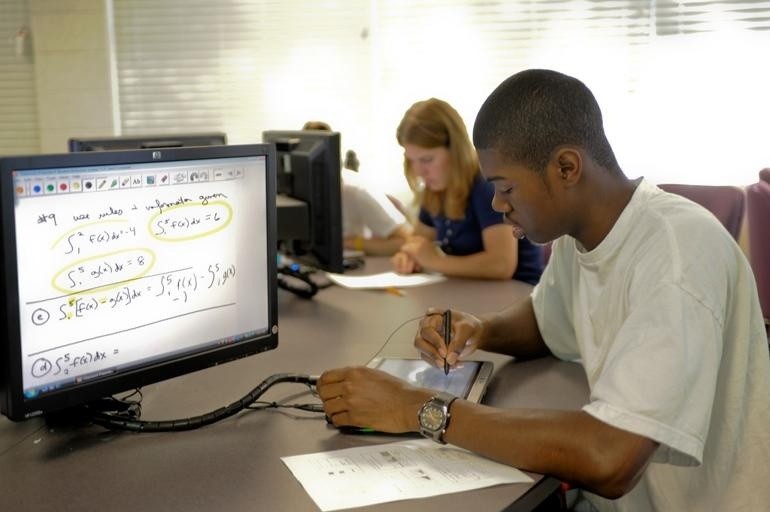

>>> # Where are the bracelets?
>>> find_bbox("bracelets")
[345,235,363,250]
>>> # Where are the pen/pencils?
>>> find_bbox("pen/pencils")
[387,195,447,258]
[444,310,451,373]
[386,287,406,298]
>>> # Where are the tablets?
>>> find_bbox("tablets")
[364,357,495,404]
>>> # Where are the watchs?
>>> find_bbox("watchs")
[417,392,460,445]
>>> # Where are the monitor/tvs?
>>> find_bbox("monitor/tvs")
[0,143,279,428]
[262,129,344,275]
[67,132,228,152]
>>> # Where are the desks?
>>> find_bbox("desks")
[0,255,592,512]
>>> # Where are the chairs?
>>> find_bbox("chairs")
[745,167,770,343]
[655,182,746,244]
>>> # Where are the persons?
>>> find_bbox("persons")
[316,70,769,512]
[304,122,415,256]
[391,98,544,286]
